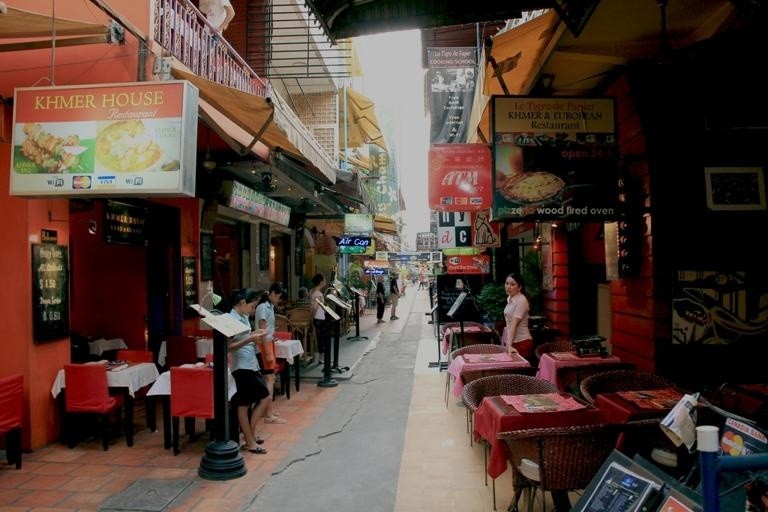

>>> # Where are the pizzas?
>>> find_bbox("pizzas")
[502,172,564,203]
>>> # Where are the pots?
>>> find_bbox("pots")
[569,334,605,345]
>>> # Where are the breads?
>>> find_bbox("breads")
[96,120,161,172]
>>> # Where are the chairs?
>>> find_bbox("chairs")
[55,286,304,400]
[117,351,154,365]
[580,369,655,408]
[497,423,619,510]
[438,316,624,434]
[463,375,561,487]
[64,363,124,452]
[168,367,217,457]
[627,411,692,459]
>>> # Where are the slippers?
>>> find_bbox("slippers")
[264,418,287,424]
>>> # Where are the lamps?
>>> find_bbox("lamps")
[533,241,549,250]
[46,212,98,234]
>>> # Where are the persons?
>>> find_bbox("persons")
[390,271,401,320]
[418,271,424,291]
[504,272,533,359]
[254,283,288,424]
[226,288,270,454]
[199,0,235,41]
[298,286,309,300]
[308,273,332,364]
[376,275,386,323]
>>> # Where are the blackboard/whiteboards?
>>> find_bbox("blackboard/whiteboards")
[200,232,213,281]
[103,199,149,247]
[259,223,269,270]
[183,256,197,319]
[31,243,70,343]
[296,231,303,276]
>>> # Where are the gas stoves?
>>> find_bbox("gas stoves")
[577,345,608,357]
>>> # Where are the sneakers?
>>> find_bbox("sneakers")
[248,447,267,454]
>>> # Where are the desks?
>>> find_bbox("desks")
[164,364,244,454]
[595,386,691,445]
[56,362,159,448]
[482,394,599,510]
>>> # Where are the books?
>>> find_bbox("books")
[568,448,704,512]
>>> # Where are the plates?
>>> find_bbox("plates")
[534,133,553,146]
[104,360,129,364]
[105,366,115,371]
[514,134,533,145]
